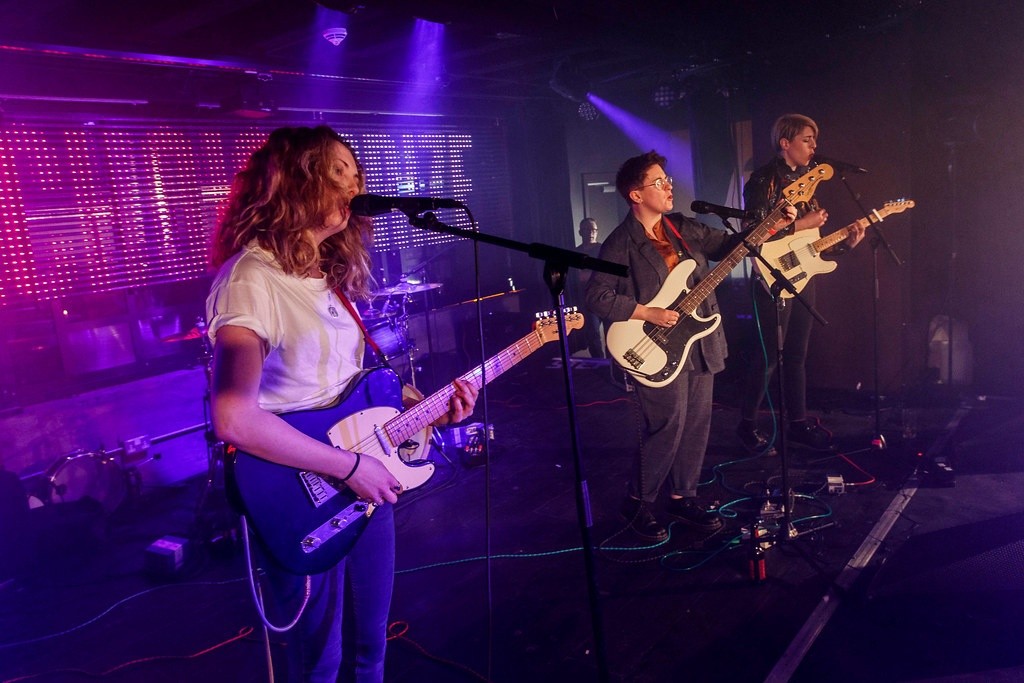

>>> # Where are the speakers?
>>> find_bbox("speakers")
[533,358,646,455]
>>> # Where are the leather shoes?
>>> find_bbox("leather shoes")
[735,424,776,456]
[664,497,721,529]
[622,497,668,541]
[779,421,832,450]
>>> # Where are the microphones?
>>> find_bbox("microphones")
[812,155,868,173]
[348,194,463,217]
[691,201,757,219]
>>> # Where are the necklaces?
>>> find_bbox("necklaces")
[290,269,339,318]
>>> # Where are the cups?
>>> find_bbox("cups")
[902,408,918,439]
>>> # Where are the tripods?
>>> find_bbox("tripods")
[699,171,930,597]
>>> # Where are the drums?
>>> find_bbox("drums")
[43,447,132,513]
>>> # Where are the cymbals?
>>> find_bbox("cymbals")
[368,283,445,297]
[159,326,211,343]
[359,309,398,321]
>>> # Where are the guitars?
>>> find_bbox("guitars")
[748,196,916,300]
[606,163,834,389]
[230,302,588,580]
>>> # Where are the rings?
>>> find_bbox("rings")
[373,499,383,507]
[666,320,670,324]
[393,480,401,491]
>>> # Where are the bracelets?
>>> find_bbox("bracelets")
[767,227,777,236]
[341,450,360,486]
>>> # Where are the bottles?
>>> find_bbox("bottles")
[750,522,759,539]
[747,544,767,587]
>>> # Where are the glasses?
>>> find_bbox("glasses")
[634,176,672,192]
[580,228,598,234]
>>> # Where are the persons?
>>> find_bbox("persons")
[567,218,611,359]
[738,112,866,455]
[207,126,482,683]
[584,149,797,542]
